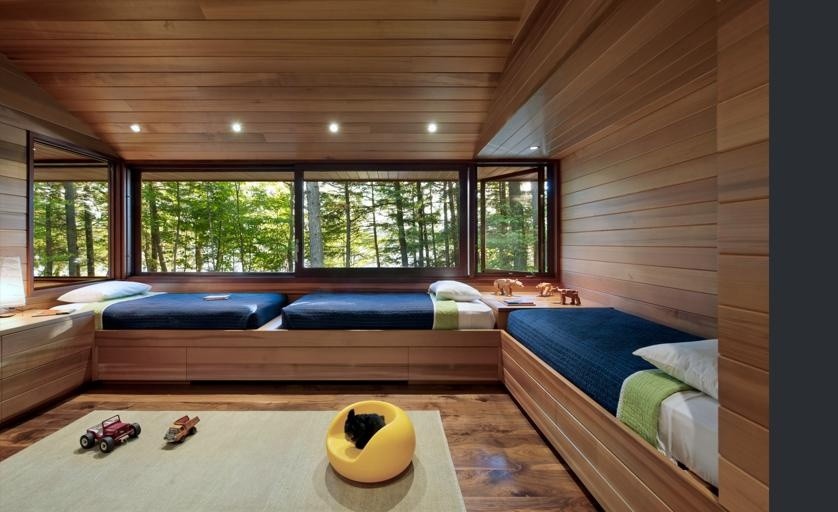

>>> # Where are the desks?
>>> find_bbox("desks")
[0,308,95,423]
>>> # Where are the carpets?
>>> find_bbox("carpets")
[1,411,468,511]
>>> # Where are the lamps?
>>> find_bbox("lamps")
[0,256,26,318]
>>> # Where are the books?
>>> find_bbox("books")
[203,295,233,301]
[501,297,535,306]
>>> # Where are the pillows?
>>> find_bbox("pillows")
[427,280,481,301]
[56,281,152,302]
